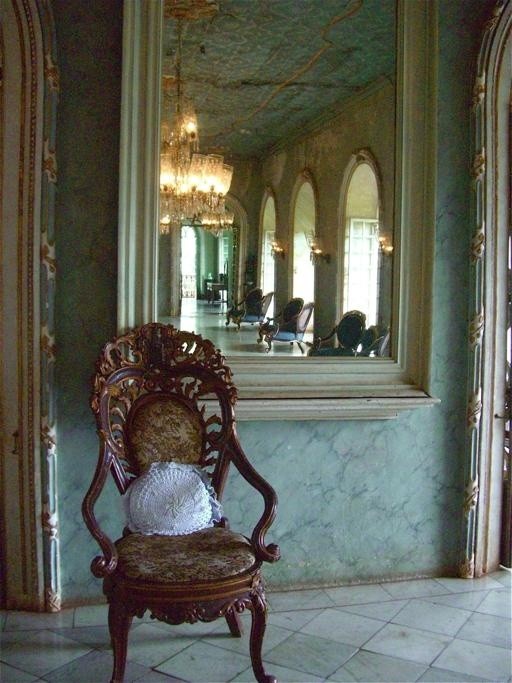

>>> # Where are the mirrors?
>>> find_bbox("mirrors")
[117,1,442,420]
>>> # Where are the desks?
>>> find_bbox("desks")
[206,282,225,304]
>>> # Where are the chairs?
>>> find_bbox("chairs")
[224,287,380,357]
[79,322,282,682]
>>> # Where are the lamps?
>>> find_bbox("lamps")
[157,0,236,237]
